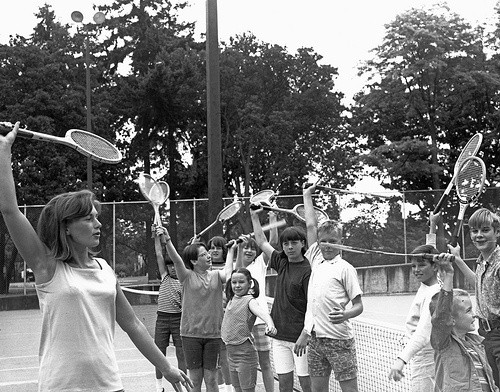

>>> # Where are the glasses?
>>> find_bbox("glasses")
[198,251,212,257]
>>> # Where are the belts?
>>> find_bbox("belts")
[479,318,499,332]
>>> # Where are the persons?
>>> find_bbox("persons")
[429,252,499,391]
[221,268,277,392]
[388,211,445,392]
[235,200,281,392]
[0,122,194,392]
[302,178,362,392]
[158,226,238,392]
[190,234,232,392]
[151,223,187,391]
[445,206,500,391]
[249,202,312,392]
